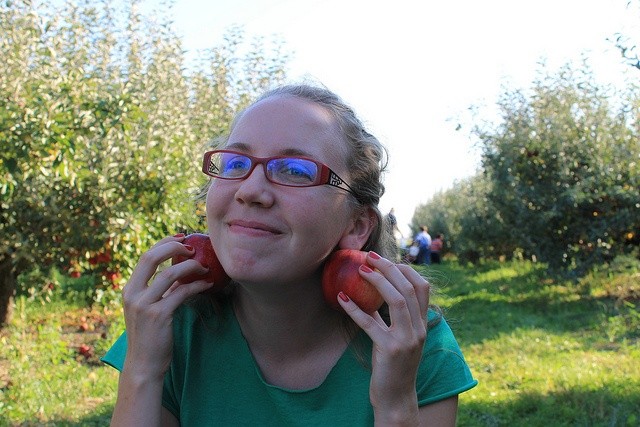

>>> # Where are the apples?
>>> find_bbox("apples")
[320,248,392,315]
[80,345,89,354]
[89,323,95,331]
[79,316,87,322]
[42,218,123,290]
[81,323,88,331]
[172,232,232,294]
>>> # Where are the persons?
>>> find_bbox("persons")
[384,208,404,254]
[414,225,433,266]
[431,233,445,264]
[98,84,477,427]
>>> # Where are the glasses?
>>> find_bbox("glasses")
[201,149,364,206]
